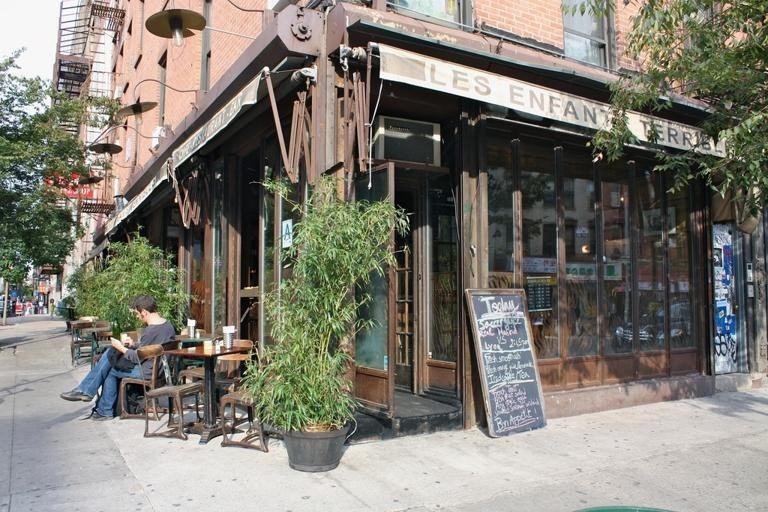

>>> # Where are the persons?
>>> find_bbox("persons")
[59,294,177,421]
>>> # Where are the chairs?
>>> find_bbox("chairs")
[70,321,284,452]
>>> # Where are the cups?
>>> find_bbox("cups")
[223,333,235,349]
[187,326,195,339]
[203,341,213,355]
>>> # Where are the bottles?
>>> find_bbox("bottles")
[215,340,220,351]
[194,329,200,338]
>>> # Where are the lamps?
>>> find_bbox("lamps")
[79,158,138,191]
[146,9,256,47]
[89,125,165,161]
[115,78,208,125]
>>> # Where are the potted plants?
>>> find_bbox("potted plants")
[239,173,411,473]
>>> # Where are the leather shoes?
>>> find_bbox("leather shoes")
[61,388,92,402]
[79,409,114,422]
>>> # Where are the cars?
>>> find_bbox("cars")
[612,291,691,348]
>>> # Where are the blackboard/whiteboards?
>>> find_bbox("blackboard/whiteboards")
[465,287,548,439]
